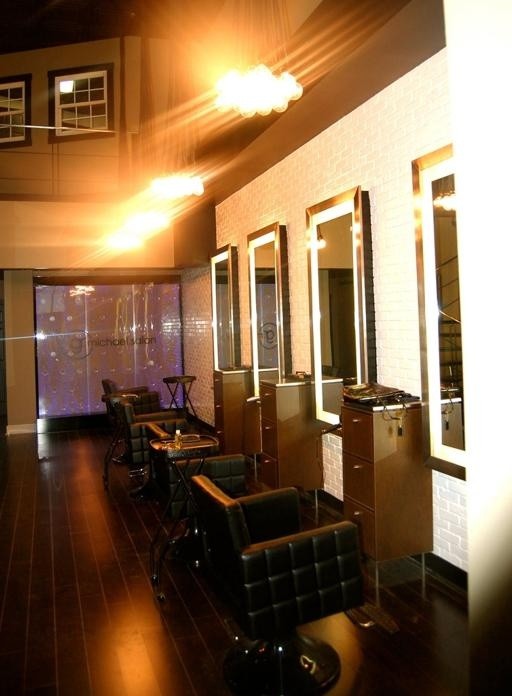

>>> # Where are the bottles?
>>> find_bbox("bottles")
[174,429,182,449]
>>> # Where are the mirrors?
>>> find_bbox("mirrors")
[307,186,376,438]
[209,244,239,369]
[412,144,466,483]
[248,221,285,401]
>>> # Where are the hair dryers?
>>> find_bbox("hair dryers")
[322,413,343,435]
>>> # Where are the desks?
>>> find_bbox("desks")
[163,375,197,418]
[146,432,219,552]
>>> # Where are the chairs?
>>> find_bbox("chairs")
[190,473,369,695]
[145,421,252,559]
[101,378,161,465]
[117,400,191,506]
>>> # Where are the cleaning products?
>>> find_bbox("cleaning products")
[175,430,184,449]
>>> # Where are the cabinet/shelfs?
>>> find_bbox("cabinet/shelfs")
[213,368,251,453]
[440,396,465,450]
[257,365,280,381]
[339,402,433,606]
